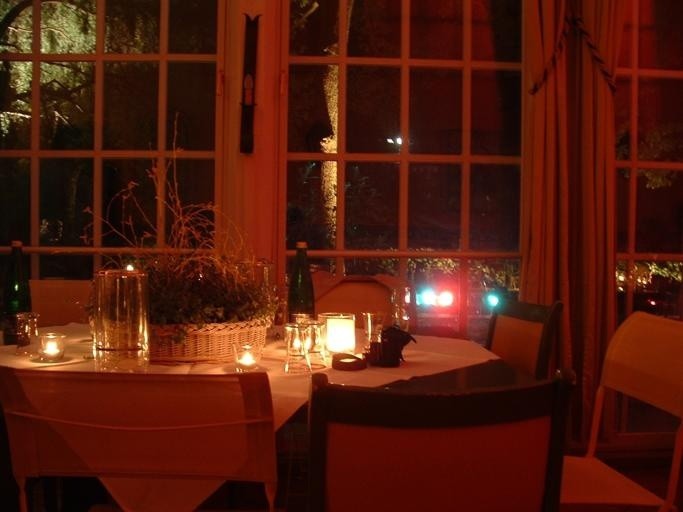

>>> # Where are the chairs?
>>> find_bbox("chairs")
[0,275,570,511]
[560,311,682,511]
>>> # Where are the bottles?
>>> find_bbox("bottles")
[287,241,315,324]
[2,239,31,345]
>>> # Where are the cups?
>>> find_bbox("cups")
[283,320,327,374]
[14,311,40,357]
[36,331,67,364]
[232,341,262,373]
[317,312,356,369]
[361,310,386,354]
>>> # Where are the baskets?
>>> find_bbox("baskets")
[88,312,274,363]
